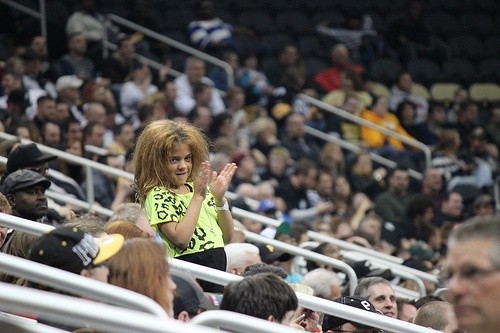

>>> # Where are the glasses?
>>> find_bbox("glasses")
[26,168,51,174]
[332,329,384,333]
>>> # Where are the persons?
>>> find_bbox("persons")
[132,118,238,295]
[22,225,125,333]
[219,272,298,325]
[106,237,178,320]
[444,212,500,333]
[1,0,500,332]
[1,169,52,249]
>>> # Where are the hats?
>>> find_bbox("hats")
[0,169,51,196]
[321,295,385,333]
[6,143,58,174]
[167,268,225,315]
[27,226,125,275]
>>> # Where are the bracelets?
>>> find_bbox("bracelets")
[214,200,230,212]
